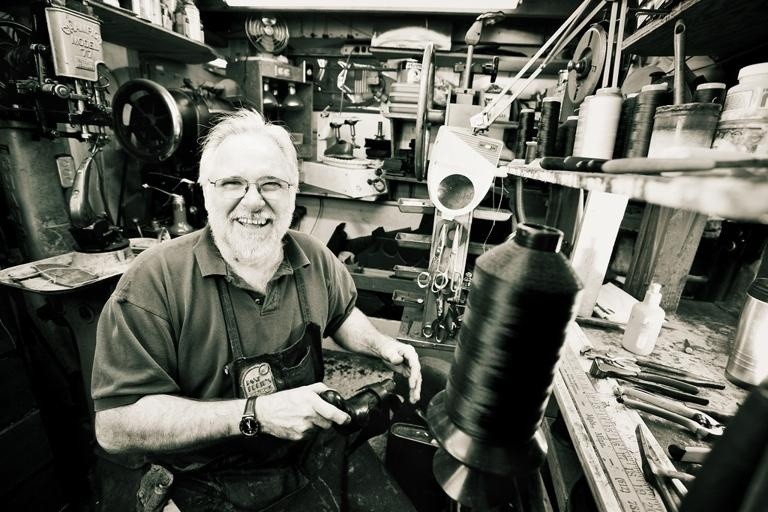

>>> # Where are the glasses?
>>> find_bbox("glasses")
[205,174,297,200]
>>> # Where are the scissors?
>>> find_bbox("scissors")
[416,223,463,343]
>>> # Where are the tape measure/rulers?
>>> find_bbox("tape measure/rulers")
[559,343,665,512]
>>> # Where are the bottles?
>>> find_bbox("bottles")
[721,62,768,111]
[570,87,624,162]
[723,276,768,392]
[168,195,194,240]
[621,282,666,357]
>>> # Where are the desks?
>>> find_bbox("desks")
[532,269,767,512]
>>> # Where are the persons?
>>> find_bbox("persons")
[92,106,423,511]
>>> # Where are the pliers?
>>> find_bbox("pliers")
[580,345,727,442]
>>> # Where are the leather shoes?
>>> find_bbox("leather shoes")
[322,140,356,160]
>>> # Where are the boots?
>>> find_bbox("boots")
[317,377,405,437]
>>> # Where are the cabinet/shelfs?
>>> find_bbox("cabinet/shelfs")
[498,1,768,235]
[230,57,315,164]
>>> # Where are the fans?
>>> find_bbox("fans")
[242,8,290,56]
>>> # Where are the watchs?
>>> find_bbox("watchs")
[238,395,261,438]
